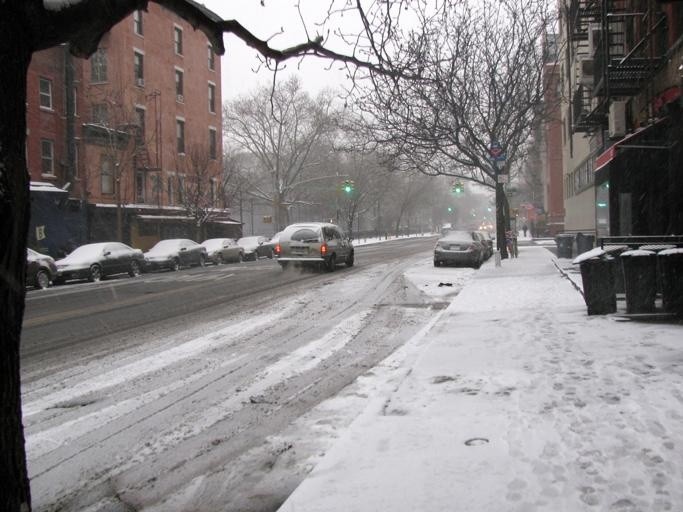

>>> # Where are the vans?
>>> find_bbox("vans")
[274,223,355,272]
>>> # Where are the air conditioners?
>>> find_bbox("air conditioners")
[177,94,183,102]
[137,78,144,87]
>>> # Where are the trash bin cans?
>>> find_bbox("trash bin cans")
[554,232,595,259]
[578,244,683,316]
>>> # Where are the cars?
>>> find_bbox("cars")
[145,239,206,271]
[201,237,244,266]
[433,229,494,266]
[53,242,143,284]
[239,237,273,261]
[26,247,57,290]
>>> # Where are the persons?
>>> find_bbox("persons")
[522,223,528,237]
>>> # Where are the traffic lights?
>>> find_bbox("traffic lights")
[342,180,355,192]
[451,180,464,193]
[447,207,453,214]
